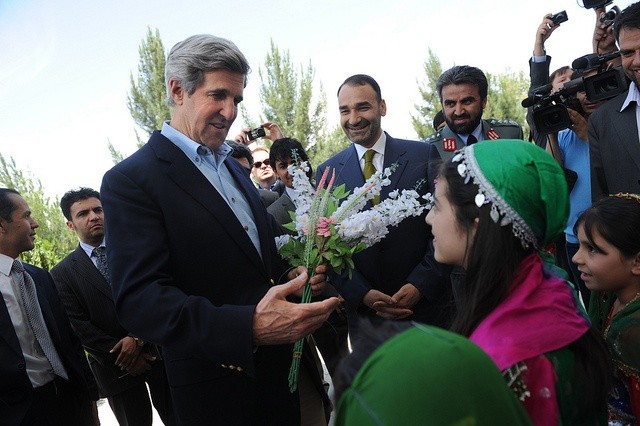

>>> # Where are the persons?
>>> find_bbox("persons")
[265,136,347,397]
[48,187,159,425]
[428,65,523,162]
[433,109,448,131]
[233,121,285,192]
[425,140,614,424]
[223,139,279,209]
[317,73,454,349]
[589,10,624,70]
[101,32,341,426]
[0,187,100,423]
[527,5,577,145]
[324,320,537,426]
[571,191,639,426]
[586,1,640,206]
[544,63,612,312]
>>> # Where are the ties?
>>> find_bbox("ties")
[10,258,69,380]
[91,245,111,286]
[361,149,382,207]
[464,131,478,145]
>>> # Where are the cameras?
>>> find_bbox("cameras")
[582,0,613,9]
[247,126,266,141]
[600,5,621,28]
[546,10,568,27]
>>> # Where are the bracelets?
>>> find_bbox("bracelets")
[130,335,144,346]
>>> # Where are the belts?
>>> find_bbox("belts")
[34,376,68,402]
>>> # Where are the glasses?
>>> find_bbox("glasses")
[252,157,270,168]
